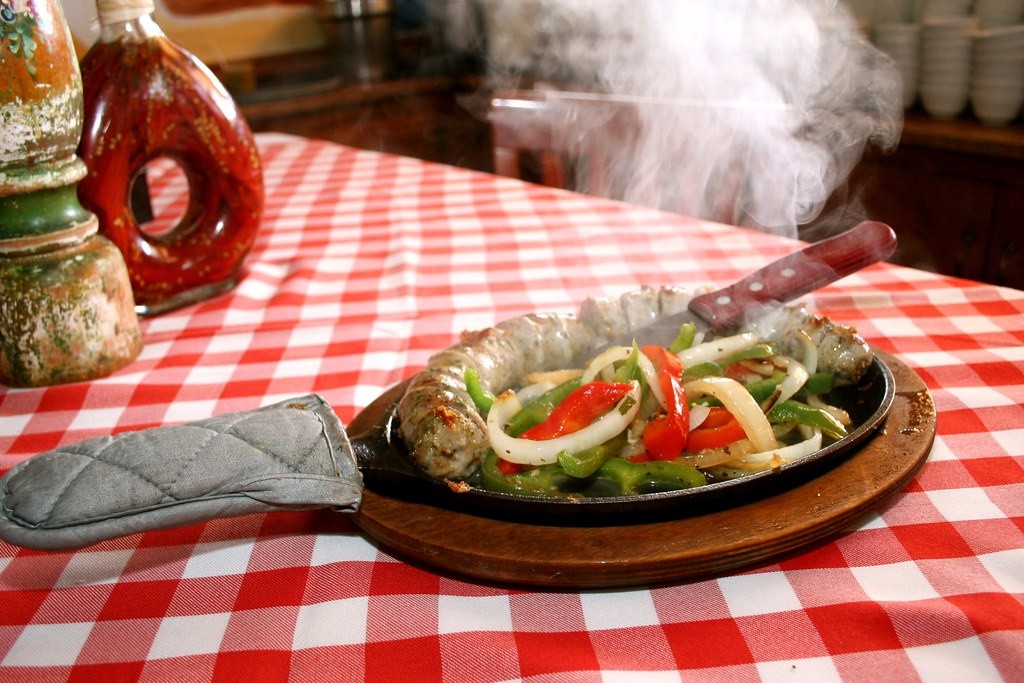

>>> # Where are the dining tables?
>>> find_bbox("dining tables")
[0,132,1024,683]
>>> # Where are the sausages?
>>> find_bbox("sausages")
[578,283,873,384]
[393,311,595,486]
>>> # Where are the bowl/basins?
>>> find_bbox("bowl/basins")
[873,0,1024,128]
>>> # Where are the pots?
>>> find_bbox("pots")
[349,355,897,529]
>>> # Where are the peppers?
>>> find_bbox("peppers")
[464,320,853,497]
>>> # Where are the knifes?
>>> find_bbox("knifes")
[567,220,899,370]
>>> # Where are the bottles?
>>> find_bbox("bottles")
[77,0,264,318]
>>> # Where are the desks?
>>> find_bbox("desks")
[486,75,1024,292]
